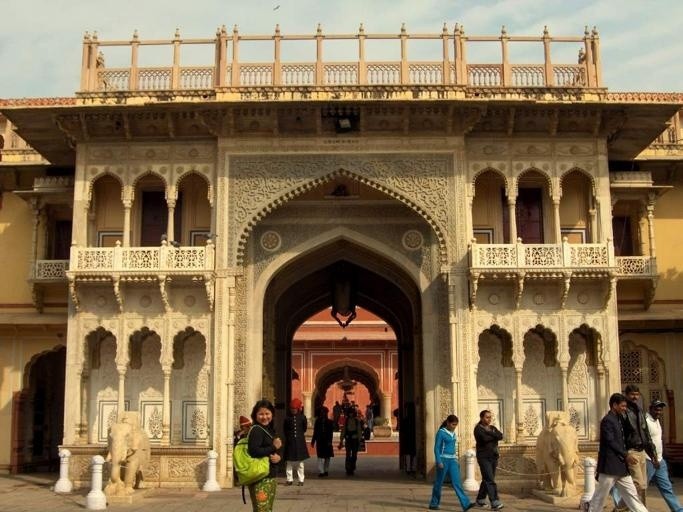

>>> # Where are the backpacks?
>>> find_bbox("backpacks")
[232,438,270,485]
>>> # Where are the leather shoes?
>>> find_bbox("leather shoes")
[464,503,475,512]
[429,506,440,510]
[297,482,305,486]
[284,482,292,486]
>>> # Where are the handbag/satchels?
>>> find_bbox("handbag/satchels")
[359,441,365,451]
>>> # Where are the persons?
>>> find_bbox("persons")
[310,405,338,477]
[233,414,253,446]
[587,393,651,512]
[611,384,661,512]
[428,414,476,512]
[361,423,370,442]
[642,399,682,511]
[332,400,341,424]
[280,398,310,488]
[353,404,364,420]
[337,406,371,477]
[472,409,505,511]
[242,396,284,512]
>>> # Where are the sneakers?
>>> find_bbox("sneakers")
[476,498,488,509]
[490,503,504,511]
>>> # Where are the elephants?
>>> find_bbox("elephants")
[535,410,586,498]
[103,420,151,497]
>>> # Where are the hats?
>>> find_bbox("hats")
[651,399,666,408]
[240,415,252,429]
